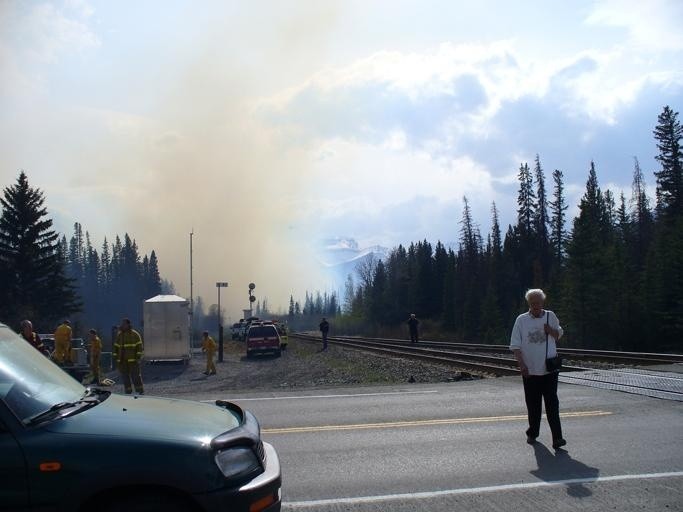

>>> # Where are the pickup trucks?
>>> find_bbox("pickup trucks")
[39,335,114,370]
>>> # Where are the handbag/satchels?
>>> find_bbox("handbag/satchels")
[546,357,562,371]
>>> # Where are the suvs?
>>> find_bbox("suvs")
[246,319,280,359]
[229,323,244,340]
[237,318,249,340]
[271,320,289,350]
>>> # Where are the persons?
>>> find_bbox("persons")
[407,313,422,343]
[110,318,146,395]
[200,330,218,376]
[509,287,567,449]
[19,320,51,358]
[319,317,330,352]
[88,328,103,385]
[54,319,75,366]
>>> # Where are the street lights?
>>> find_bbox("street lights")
[187,226,197,346]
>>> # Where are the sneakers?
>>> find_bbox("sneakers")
[553,437,566,448]
[210,371,216,375]
[202,371,209,375]
[527,435,536,444]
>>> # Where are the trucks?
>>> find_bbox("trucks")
[46,347,90,384]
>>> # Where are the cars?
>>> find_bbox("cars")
[0,322,282,512]
